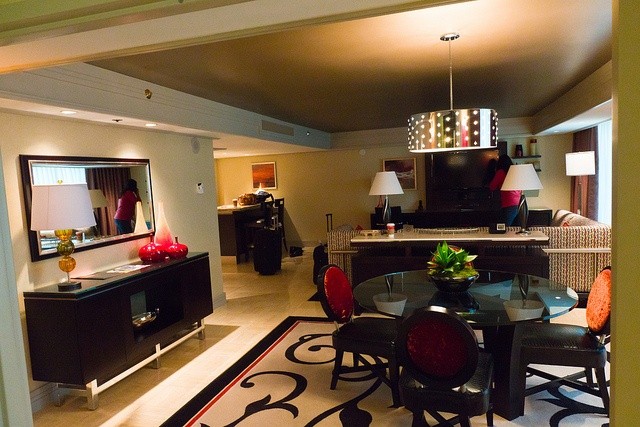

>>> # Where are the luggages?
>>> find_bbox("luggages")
[313,214,333,284]
[254,215,281,275]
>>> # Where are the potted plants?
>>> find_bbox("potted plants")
[425,240,481,292]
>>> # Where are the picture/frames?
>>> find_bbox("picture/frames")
[251,160,277,189]
[382,157,418,191]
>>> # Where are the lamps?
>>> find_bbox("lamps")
[500,163,543,235]
[28,178,97,291]
[373,275,407,315]
[406,31,499,153]
[564,149,597,216]
[503,265,544,321]
[86,187,109,208]
[367,170,404,233]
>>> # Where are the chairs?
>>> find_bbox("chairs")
[429,244,474,268]
[316,263,402,407]
[519,265,613,417]
[391,306,498,426]
[234,199,274,264]
[273,198,287,253]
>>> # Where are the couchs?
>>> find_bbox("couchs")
[326,209,611,293]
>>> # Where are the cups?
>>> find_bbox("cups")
[386,223,396,239]
[233,199,237,207]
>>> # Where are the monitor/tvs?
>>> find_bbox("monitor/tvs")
[432,151,500,197]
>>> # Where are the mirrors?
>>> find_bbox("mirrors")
[18,151,157,262]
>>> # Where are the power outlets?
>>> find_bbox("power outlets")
[196,182,205,193]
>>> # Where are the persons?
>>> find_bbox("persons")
[489,154,522,226]
[114,178,142,234]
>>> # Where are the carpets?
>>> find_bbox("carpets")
[158,313,611,426]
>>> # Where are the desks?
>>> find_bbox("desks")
[352,264,581,356]
[217,196,288,264]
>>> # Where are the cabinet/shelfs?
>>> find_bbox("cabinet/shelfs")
[349,230,550,315]
[370,208,553,225]
[22,249,220,410]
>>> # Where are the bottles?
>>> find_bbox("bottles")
[530,138,537,156]
[515,144,523,157]
